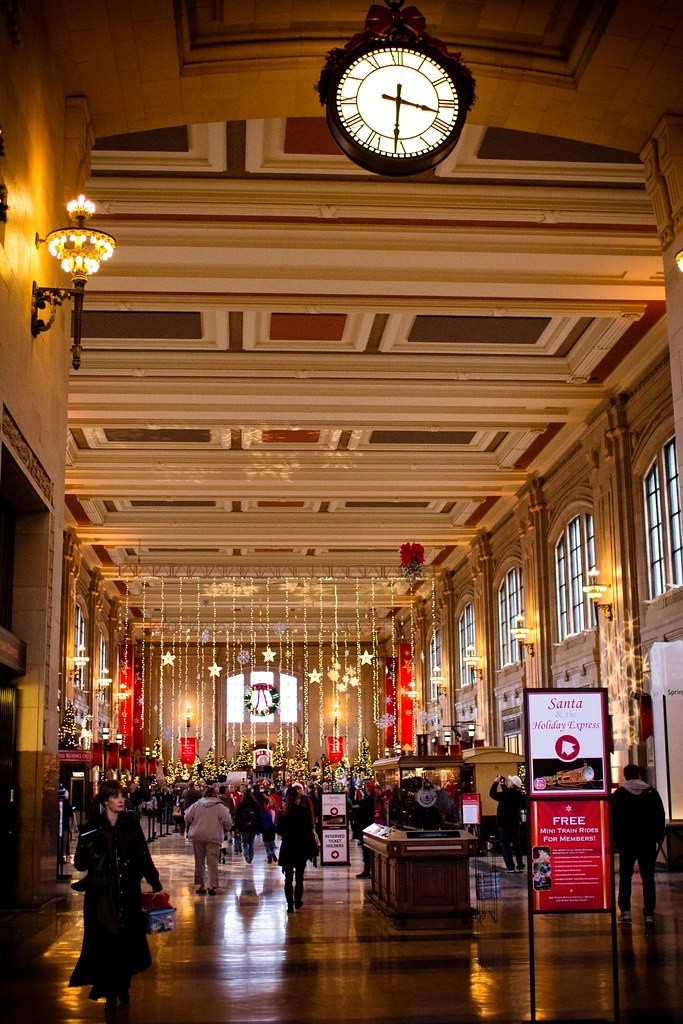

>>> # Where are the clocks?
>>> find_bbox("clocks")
[313,0,478,177]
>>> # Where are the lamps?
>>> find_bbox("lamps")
[96,668,112,706]
[405,680,420,709]
[463,643,483,681]
[70,645,90,689]
[511,614,534,658]
[460,721,481,748]
[183,700,193,731]
[96,725,118,783]
[152,750,157,776]
[581,567,613,621]
[31,194,117,371]
[429,665,446,697]
[396,745,402,756]
[145,746,150,776]
[443,731,452,746]
[332,703,341,728]
[115,683,129,711]
[112,732,128,782]
[385,748,390,759]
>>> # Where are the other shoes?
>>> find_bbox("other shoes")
[504,867,525,874]
[356,868,371,879]
[196,887,207,894]
[209,888,214,895]
[646,915,656,926]
[104,995,117,1016]
[296,900,303,910]
[618,910,632,922]
[286,904,294,913]
[243,852,252,864]
[272,852,277,862]
[267,853,273,864]
[117,987,130,1003]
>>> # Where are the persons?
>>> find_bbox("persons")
[185,786,231,895]
[59,783,78,864]
[612,764,665,924]
[490,774,525,873]
[152,775,384,878]
[276,787,313,913]
[68,780,163,1024]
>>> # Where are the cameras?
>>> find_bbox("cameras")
[500,776,505,782]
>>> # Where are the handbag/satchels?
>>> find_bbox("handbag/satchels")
[307,831,319,859]
[88,821,109,878]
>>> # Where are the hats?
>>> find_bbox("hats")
[292,782,304,792]
[508,774,523,789]
[368,781,375,792]
